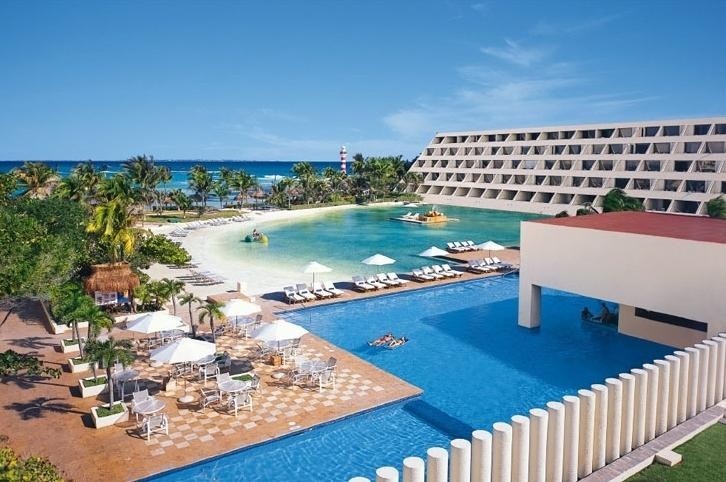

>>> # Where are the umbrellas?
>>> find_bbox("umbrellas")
[149,336,216,396]
[299,260,333,294]
[478,240,505,257]
[219,298,262,345]
[416,245,451,266]
[250,317,309,351]
[359,252,396,274]
[125,309,183,342]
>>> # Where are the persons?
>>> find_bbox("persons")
[384,336,406,349]
[367,331,393,347]
[599,302,609,324]
[580,305,594,321]
[252,229,264,241]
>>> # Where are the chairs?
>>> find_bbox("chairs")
[352,273,410,293]
[171,241,224,284]
[172,214,251,237]
[111,315,336,441]
[447,240,480,254]
[468,256,513,274]
[406,213,419,220]
[400,211,412,218]
[412,264,464,283]
[284,280,344,305]
